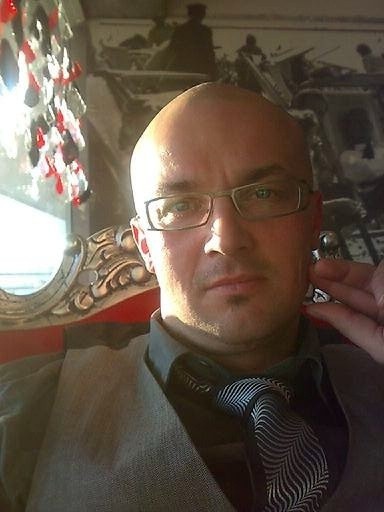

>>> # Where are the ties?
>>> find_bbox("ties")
[168,359,333,512]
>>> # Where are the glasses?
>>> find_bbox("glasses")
[135,179,314,232]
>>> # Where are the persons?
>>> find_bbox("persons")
[0,81,384,509]
